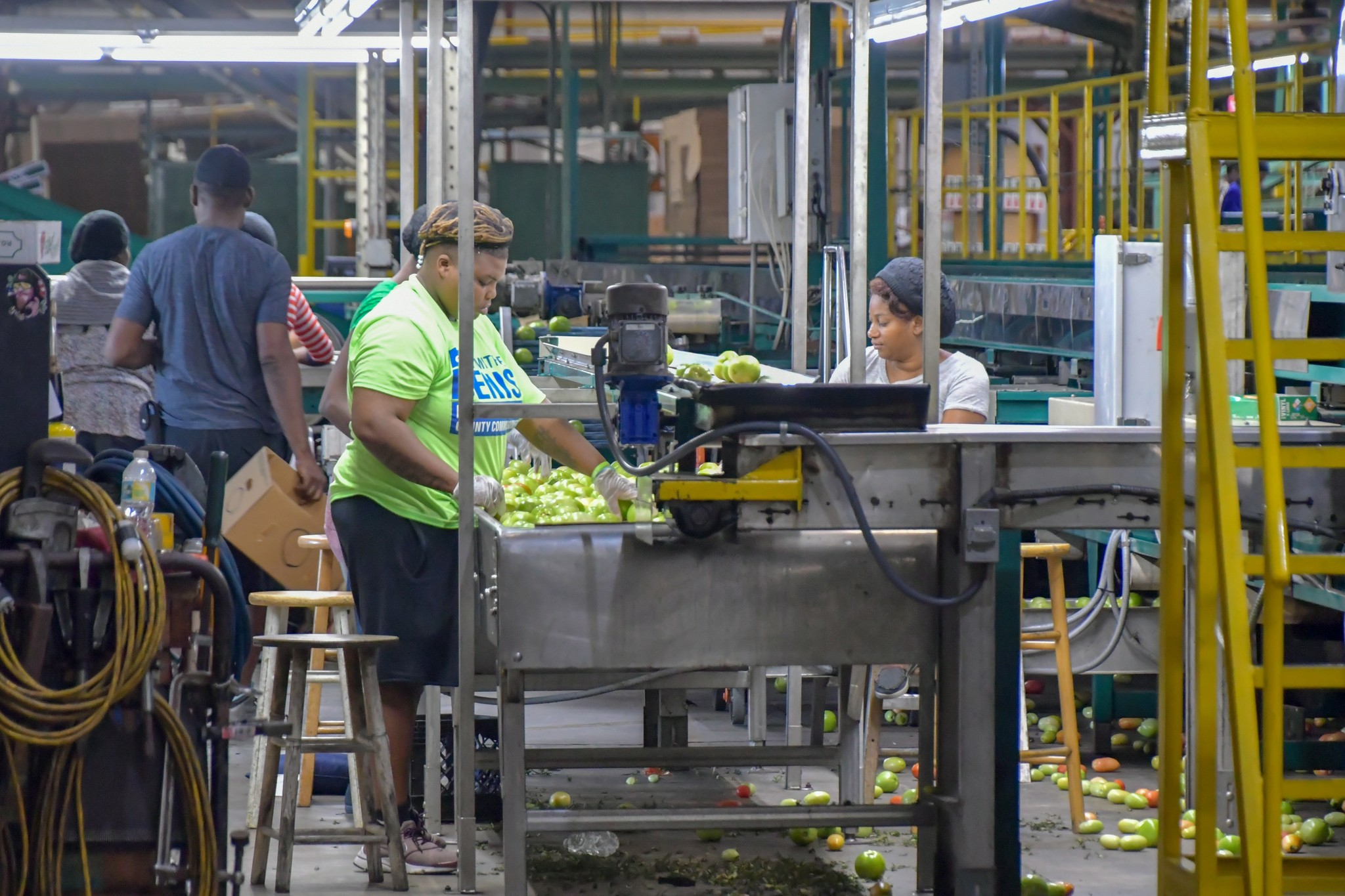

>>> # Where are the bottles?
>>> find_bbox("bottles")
[563,830,620,857]
[119,450,157,534]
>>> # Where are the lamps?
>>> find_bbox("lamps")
[294,0,380,35]
[849,0,1057,44]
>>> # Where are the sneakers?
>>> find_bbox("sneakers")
[351,819,458,875]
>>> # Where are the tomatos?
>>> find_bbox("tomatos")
[529,321,544,328]
[1020,873,1075,896]
[515,326,535,341]
[549,316,571,332]
[617,757,918,896]
[894,712,908,726]
[1021,591,1161,609]
[492,459,723,528]
[884,710,895,722]
[676,362,712,384]
[712,350,761,384]
[1024,674,1345,857]
[548,790,572,808]
[823,710,837,733]
[774,677,787,693]
[667,344,674,366]
[568,419,585,436]
[515,348,533,362]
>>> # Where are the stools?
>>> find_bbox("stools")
[1018,543,1089,834]
[251,634,411,895]
[244,592,365,829]
[296,535,337,807]
[857,664,941,804]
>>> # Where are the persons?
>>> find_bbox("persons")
[830,256,991,425]
[101,144,326,725]
[236,209,332,366]
[316,200,458,814]
[1219,159,1270,214]
[50,209,156,476]
[331,199,652,875]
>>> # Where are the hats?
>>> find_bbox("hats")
[403,199,464,254]
[877,256,956,338]
[417,196,516,270]
[71,209,130,265]
[238,211,278,250]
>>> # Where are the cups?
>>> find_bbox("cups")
[150,512,175,554]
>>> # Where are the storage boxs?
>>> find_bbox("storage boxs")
[225,446,346,595]
[2,219,61,266]
